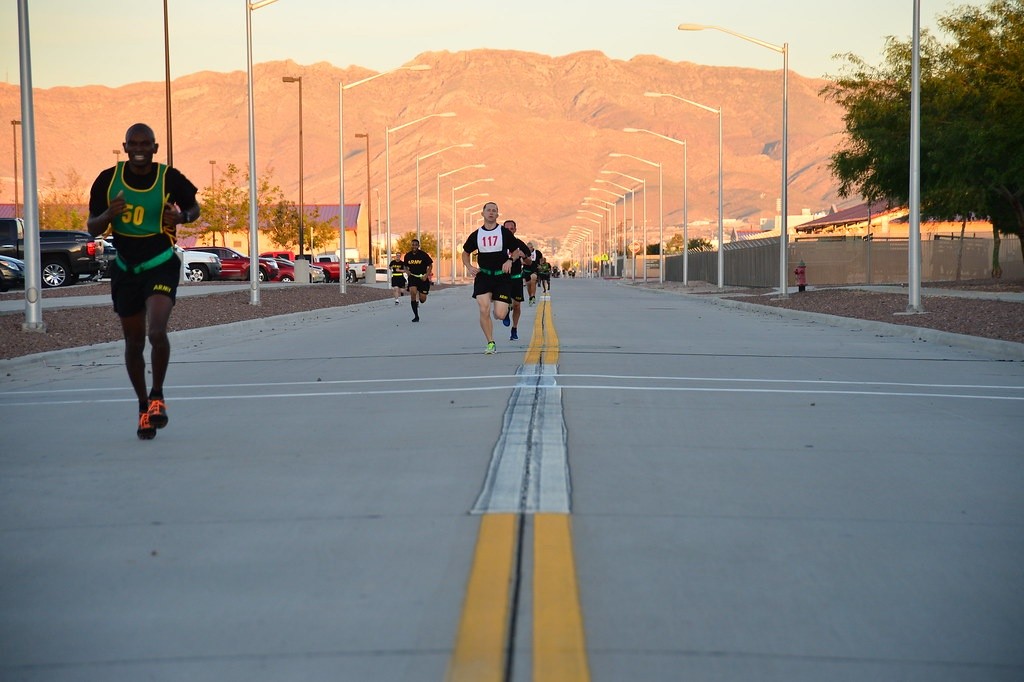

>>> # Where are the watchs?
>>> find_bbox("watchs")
[508,258,513,263]
[522,255,527,260]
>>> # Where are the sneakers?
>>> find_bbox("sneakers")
[503,306,511,327]
[412,317,419,322]
[510,332,519,340]
[485,342,497,355]
[136,411,157,439]
[147,397,167,429]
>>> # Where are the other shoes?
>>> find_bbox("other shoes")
[532,297,536,303]
[529,300,534,306]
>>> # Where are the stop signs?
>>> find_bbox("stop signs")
[629,242,640,253]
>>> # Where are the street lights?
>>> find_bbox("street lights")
[451,178,502,283]
[594,179,635,282]
[609,153,663,283]
[415,143,474,250]
[385,112,457,290]
[565,197,618,277]
[590,188,626,279]
[437,164,486,284]
[624,128,688,285]
[283,76,310,283]
[644,92,724,288]
[339,65,432,294]
[678,23,789,295]
[11,120,22,219]
[354,133,376,284]
[601,171,647,282]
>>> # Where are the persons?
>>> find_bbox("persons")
[389,252,406,305]
[522,242,576,305]
[404,239,433,322]
[87,123,200,440]
[502,220,531,342]
[461,202,519,356]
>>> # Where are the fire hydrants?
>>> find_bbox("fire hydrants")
[793,260,808,292]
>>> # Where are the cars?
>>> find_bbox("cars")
[0,217,222,292]
[184,246,388,282]
